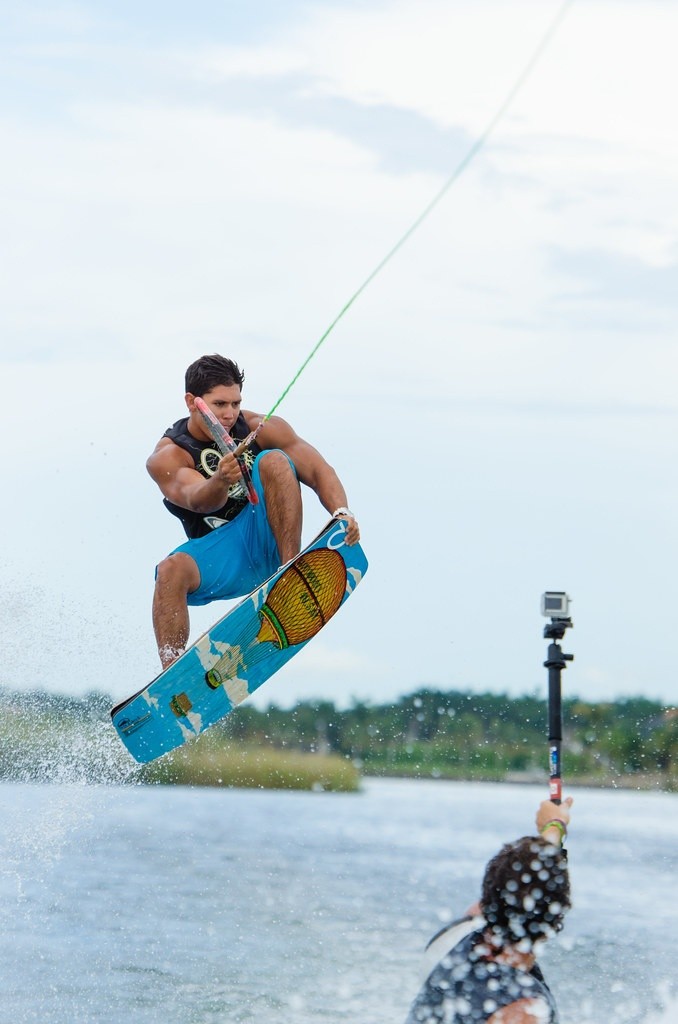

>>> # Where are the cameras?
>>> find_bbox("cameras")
[542,594,570,617]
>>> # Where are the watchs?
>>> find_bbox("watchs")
[332,507,354,517]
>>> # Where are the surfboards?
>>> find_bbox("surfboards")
[109,515,370,763]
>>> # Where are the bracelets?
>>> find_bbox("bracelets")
[542,818,568,843]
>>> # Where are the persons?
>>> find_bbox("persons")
[404,795,573,1024]
[146,354,361,671]
